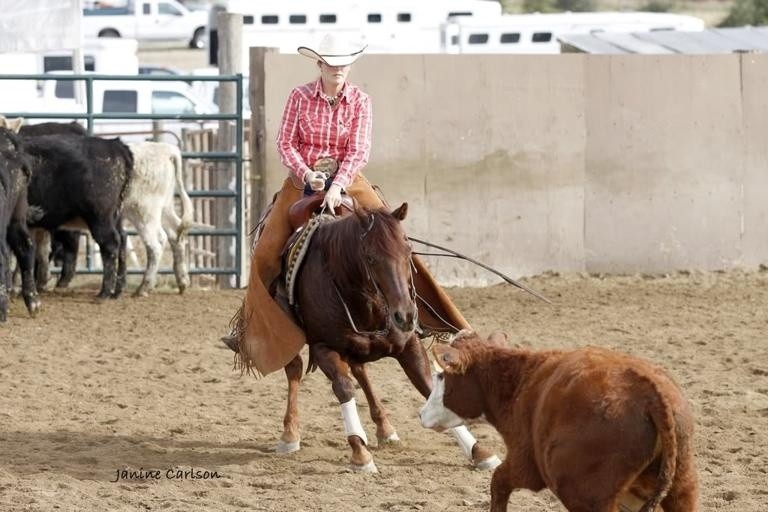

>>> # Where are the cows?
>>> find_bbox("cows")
[416,327,703,512]
[0,115,195,323]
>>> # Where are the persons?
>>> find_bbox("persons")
[223,32,383,354]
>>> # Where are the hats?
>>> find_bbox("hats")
[297,43,369,67]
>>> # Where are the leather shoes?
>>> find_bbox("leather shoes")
[223,337,238,353]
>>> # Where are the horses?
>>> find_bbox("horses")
[272,195,505,476]
